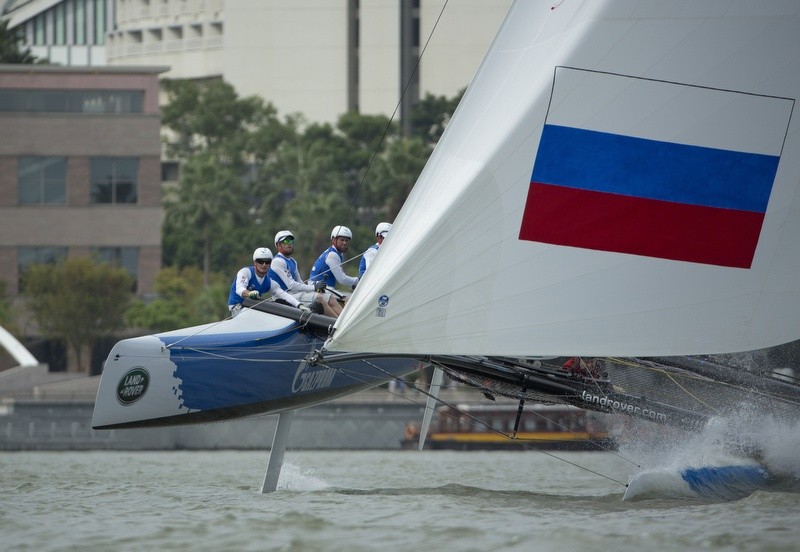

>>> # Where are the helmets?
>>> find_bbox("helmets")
[331,225,352,240]
[253,248,273,262]
[375,222,392,237]
[275,231,294,248]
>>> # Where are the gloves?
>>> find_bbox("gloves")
[299,305,310,311]
[248,291,260,299]
[315,280,326,289]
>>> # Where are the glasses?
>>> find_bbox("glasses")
[279,239,293,245]
[255,259,272,264]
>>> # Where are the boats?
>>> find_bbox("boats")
[401,401,660,452]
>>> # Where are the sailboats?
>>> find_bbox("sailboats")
[92,0,800,502]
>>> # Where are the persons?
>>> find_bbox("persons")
[561,357,625,394]
[308,225,359,306]
[269,229,343,319]
[359,221,393,279]
[226,247,312,318]
